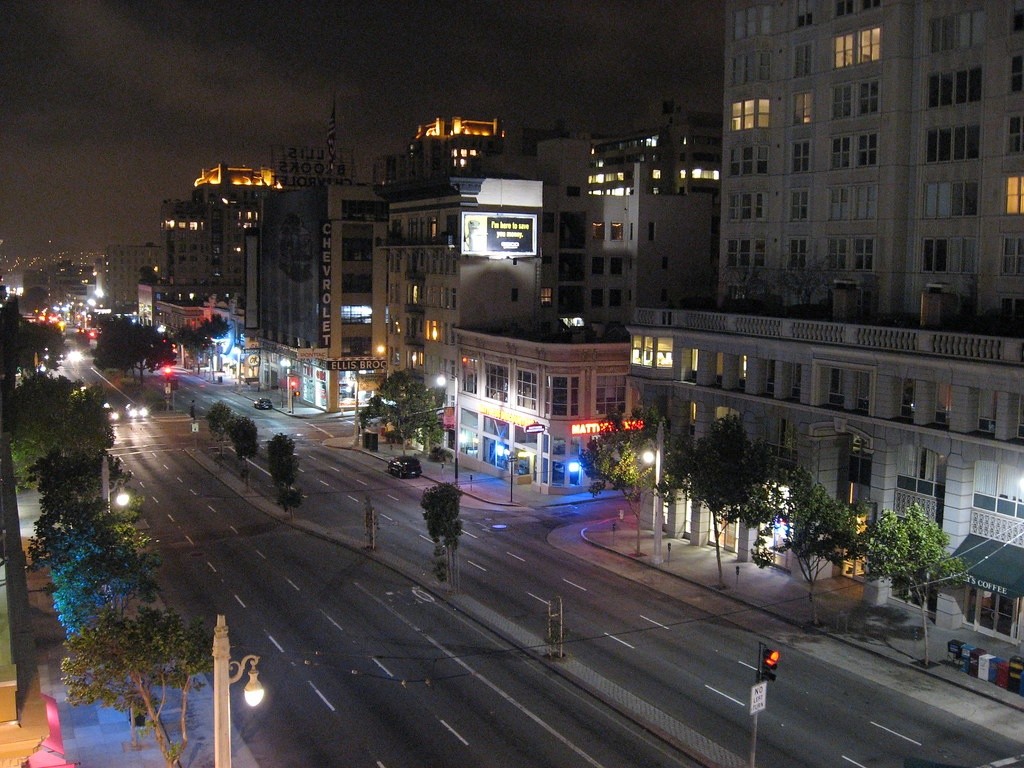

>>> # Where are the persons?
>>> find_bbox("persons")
[190,400,195,419]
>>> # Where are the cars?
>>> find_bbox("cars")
[252,397,273,410]
[126,403,149,418]
[68,350,84,361]
[388,455,421,478]
[102,403,120,422]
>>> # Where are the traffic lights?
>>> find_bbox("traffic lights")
[88,332,95,337]
[164,366,171,378]
[295,391,300,396]
[290,380,296,388]
[759,643,779,682]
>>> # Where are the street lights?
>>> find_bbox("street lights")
[641,420,666,565]
[211,615,266,768]
[348,380,361,445]
[102,455,131,520]
[232,346,243,393]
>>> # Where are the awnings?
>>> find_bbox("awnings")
[939,534,1024,599]
